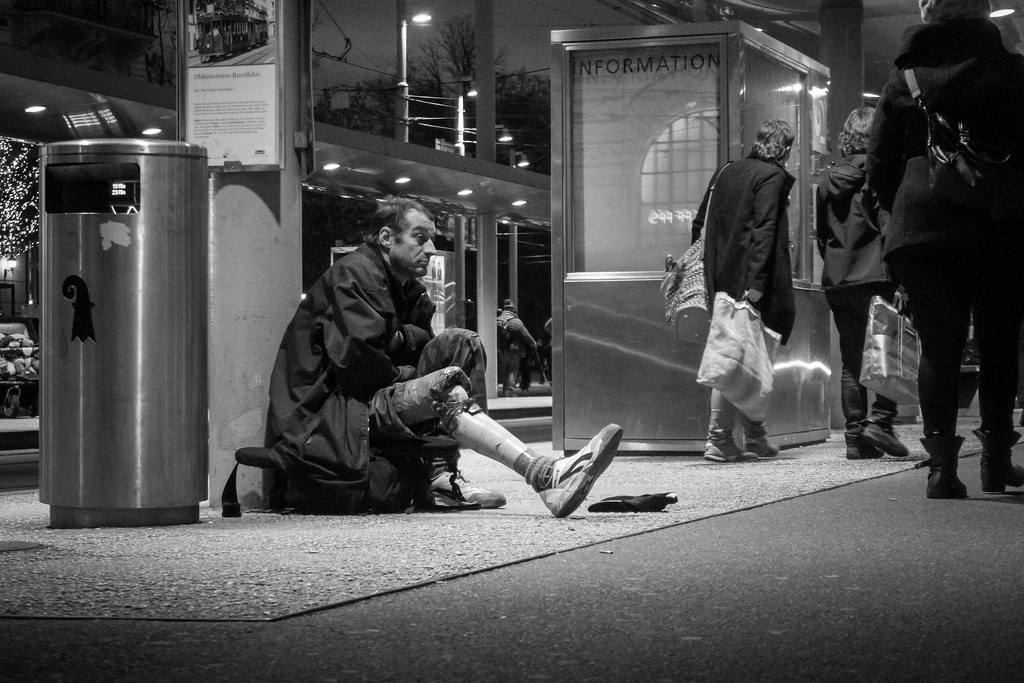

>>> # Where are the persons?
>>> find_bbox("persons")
[545,316,553,346]
[267,198,624,517]
[494,298,537,396]
[865,0,1024,498]
[815,106,909,461]
[691,120,794,463]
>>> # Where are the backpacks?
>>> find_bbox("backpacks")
[495,316,520,350]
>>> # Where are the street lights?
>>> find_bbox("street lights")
[509,150,529,317]
[396,2,431,142]
[454,75,477,328]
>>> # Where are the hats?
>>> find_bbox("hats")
[504,299,518,314]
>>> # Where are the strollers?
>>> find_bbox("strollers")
[0,315,41,418]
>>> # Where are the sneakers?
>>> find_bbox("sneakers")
[415,467,507,509]
[538,423,623,519]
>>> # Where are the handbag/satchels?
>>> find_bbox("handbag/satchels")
[696,291,784,423]
[221,430,416,519]
[859,296,923,407]
[920,111,999,209]
[660,238,714,345]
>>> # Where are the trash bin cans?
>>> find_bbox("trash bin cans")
[37,140,209,527]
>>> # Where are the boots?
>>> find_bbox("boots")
[914,435,967,498]
[972,427,1024,493]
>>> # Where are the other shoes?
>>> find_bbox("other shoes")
[844,432,885,459]
[503,389,517,397]
[703,441,759,462]
[743,442,779,456]
[862,424,908,457]
